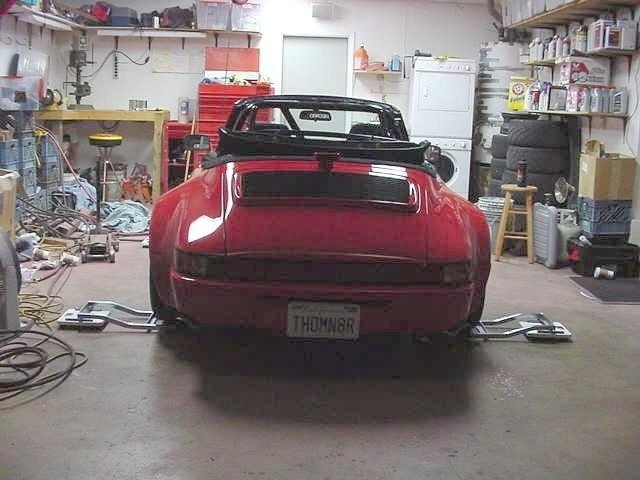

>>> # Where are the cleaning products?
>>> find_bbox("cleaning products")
[388,53,401,71]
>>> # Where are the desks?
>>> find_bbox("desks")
[36,110,170,204]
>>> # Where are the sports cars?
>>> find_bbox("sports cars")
[143,92,497,355]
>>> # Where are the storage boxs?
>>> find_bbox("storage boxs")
[0,168,21,254]
[566,152,640,278]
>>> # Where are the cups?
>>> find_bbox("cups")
[32,248,50,260]
[594,266,615,281]
[60,252,80,266]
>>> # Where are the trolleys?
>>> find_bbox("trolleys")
[74,231,123,263]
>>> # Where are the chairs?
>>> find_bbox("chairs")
[252,123,291,137]
[346,124,385,143]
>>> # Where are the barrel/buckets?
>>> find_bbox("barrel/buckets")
[477,197,515,251]
[527,24,589,60]
[524,79,628,112]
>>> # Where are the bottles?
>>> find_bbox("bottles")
[543,33,570,58]
[516,157,527,186]
[178,96,189,124]
[389,52,402,72]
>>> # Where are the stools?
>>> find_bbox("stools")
[495,184,538,263]
[87,133,129,201]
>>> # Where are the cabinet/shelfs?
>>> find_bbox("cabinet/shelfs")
[163,121,194,194]
[506,0,640,119]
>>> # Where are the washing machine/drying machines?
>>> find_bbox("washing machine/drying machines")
[409,55,477,139]
[410,136,473,202]
